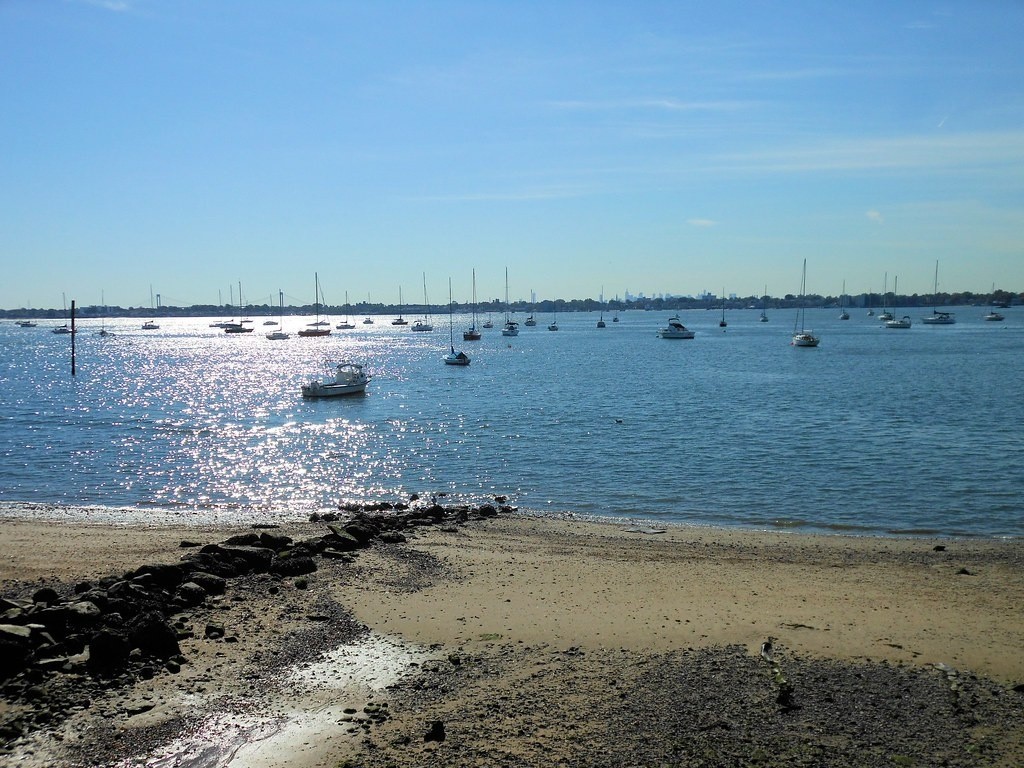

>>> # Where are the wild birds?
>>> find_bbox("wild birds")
[759,635,780,658]
[432,495,437,505]
[616,419,623,422]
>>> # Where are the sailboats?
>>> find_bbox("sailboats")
[986,282,1003,322]
[209,281,254,334]
[791,257,819,347]
[262,294,278,325]
[93,289,114,336]
[837,260,958,329]
[463,268,482,341]
[363,291,374,325]
[484,268,559,336]
[141,284,159,329]
[596,285,620,328]
[443,278,471,365]
[297,272,331,336]
[336,292,356,329]
[266,295,290,340]
[52,293,76,333]
[391,286,409,325]
[20,300,37,327]
[411,272,434,331]
[760,282,770,323]
[720,286,728,328]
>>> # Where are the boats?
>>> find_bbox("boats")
[300,363,371,400]
[655,317,697,341]
